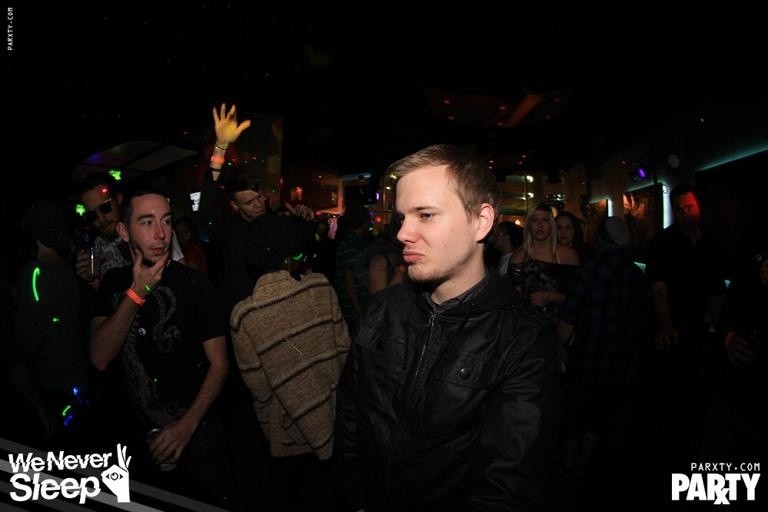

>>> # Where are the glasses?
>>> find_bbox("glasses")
[86,197,112,222]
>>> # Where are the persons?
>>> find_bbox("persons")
[1,103,764,508]
